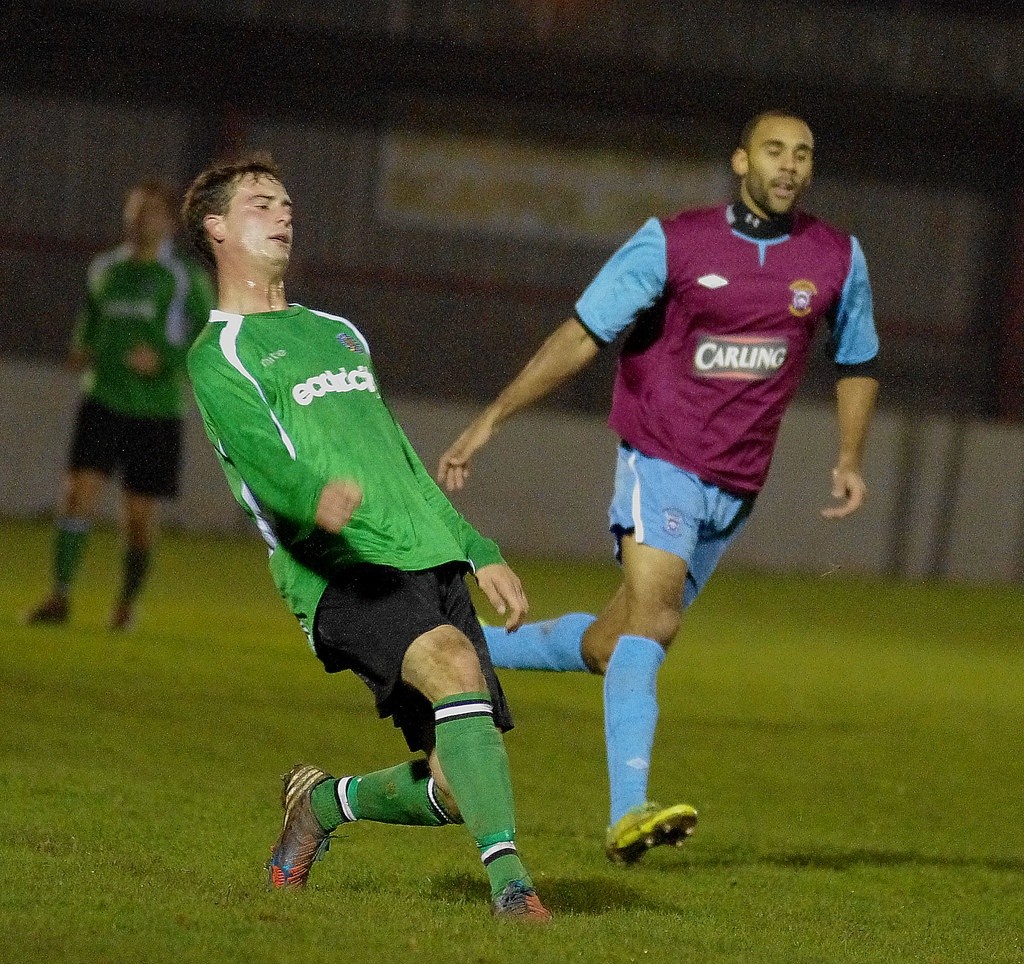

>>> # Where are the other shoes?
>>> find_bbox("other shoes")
[113,604,134,634]
[28,596,68,628]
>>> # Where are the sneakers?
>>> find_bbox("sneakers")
[478,616,489,628]
[605,804,697,866]
[268,761,336,889]
[490,885,553,924]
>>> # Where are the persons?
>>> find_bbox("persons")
[22,178,215,631]
[439,104,880,861]
[180,150,547,924]
